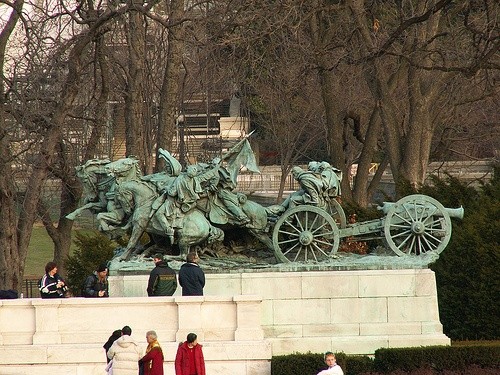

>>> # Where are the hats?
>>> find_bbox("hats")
[98,264,107,272]
[151,253,163,260]
[44,262,56,272]
[187,334,197,343]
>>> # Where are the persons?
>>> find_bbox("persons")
[317,352,344,375]
[108,326,139,375]
[175,333,206,375]
[139,148,342,245]
[178,252,205,296]
[103,329,122,364]
[37,262,68,299]
[138,331,164,375]
[82,264,109,298]
[146,253,177,296]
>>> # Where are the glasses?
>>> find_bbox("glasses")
[193,257,198,260]
